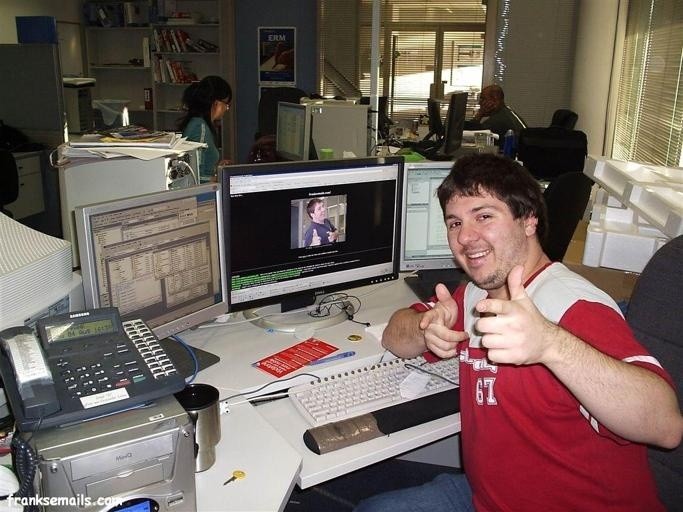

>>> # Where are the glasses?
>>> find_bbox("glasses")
[216,99,233,112]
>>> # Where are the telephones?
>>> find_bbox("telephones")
[0,306,185,433]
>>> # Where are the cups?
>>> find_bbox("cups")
[397,128,404,135]
[474,131,493,153]
[318,149,334,160]
[412,119,420,134]
[173,383,222,475]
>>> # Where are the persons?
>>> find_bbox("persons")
[462,85,528,159]
[301,197,339,246]
[348,148,683,512]
[174,74,233,186]
[270,40,293,69]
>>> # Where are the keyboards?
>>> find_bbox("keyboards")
[287,355,460,428]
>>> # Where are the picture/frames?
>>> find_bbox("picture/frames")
[258,86,279,102]
[257,26,297,86]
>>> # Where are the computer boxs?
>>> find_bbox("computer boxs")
[64,86,92,134]
[302,104,376,161]
[21,394,197,512]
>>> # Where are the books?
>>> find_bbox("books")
[74,1,221,147]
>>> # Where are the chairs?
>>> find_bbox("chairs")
[255,88,318,163]
[549,110,578,131]
[539,172,591,262]
[626,236,683,512]
[517,127,587,179]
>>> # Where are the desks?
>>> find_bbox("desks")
[582,153,683,275]
[0,278,461,512]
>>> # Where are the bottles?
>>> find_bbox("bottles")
[503,129,516,160]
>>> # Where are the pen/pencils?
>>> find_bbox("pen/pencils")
[309,352,357,366]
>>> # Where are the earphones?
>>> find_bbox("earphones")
[346,313,354,320]
[342,303,352,312]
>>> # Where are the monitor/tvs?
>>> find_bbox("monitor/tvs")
[217,156,405,333]
[423,98,443,140]
[425,92,468,155]
[360,96,392,139]
[275,101,311,161]
[74,181,229,341]
[399,161,461,273]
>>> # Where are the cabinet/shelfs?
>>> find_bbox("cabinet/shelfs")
[152,23,219,132]
[3,151,46,220]
[83,25,152,114]
